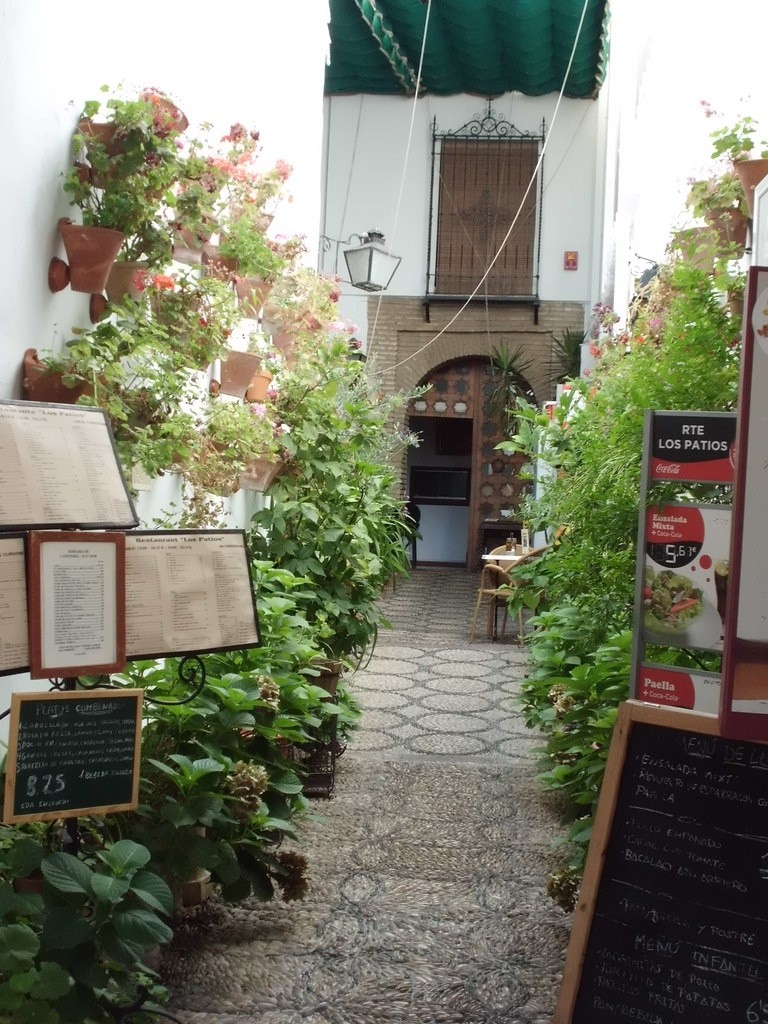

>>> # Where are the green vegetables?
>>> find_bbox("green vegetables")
[645,566,704,634]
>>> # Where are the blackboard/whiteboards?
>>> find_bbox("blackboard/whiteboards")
[552,698,768,1023]
[3,689,144,824]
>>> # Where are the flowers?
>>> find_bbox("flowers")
[684,100,768,218]
[65,78,364,459]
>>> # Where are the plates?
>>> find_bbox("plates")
[643,599,723,649]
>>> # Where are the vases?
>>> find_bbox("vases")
[57,223,127,296]
[106,260,149,304]
[272,324,298,352]
[238,209,275,235]
[234,276,274,319]
[705,208,748,259]
[152,292,200,339]
[728,289,744,316]
[199,244,236,283]
[79,120,124,190]
[171,205,220,269]
[190,329,214,369]
[27,348,284,492]
[734,159,768,216]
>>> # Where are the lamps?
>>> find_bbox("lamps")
[320,230,403,292]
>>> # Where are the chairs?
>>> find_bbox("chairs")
[469,543,556,648]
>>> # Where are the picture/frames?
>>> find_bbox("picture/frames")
[675,226,715,272]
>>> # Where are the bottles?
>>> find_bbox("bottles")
[505,532,517,552]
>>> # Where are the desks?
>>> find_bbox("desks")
[481,553,541,640]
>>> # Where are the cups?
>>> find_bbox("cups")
[715,559,729,644]
